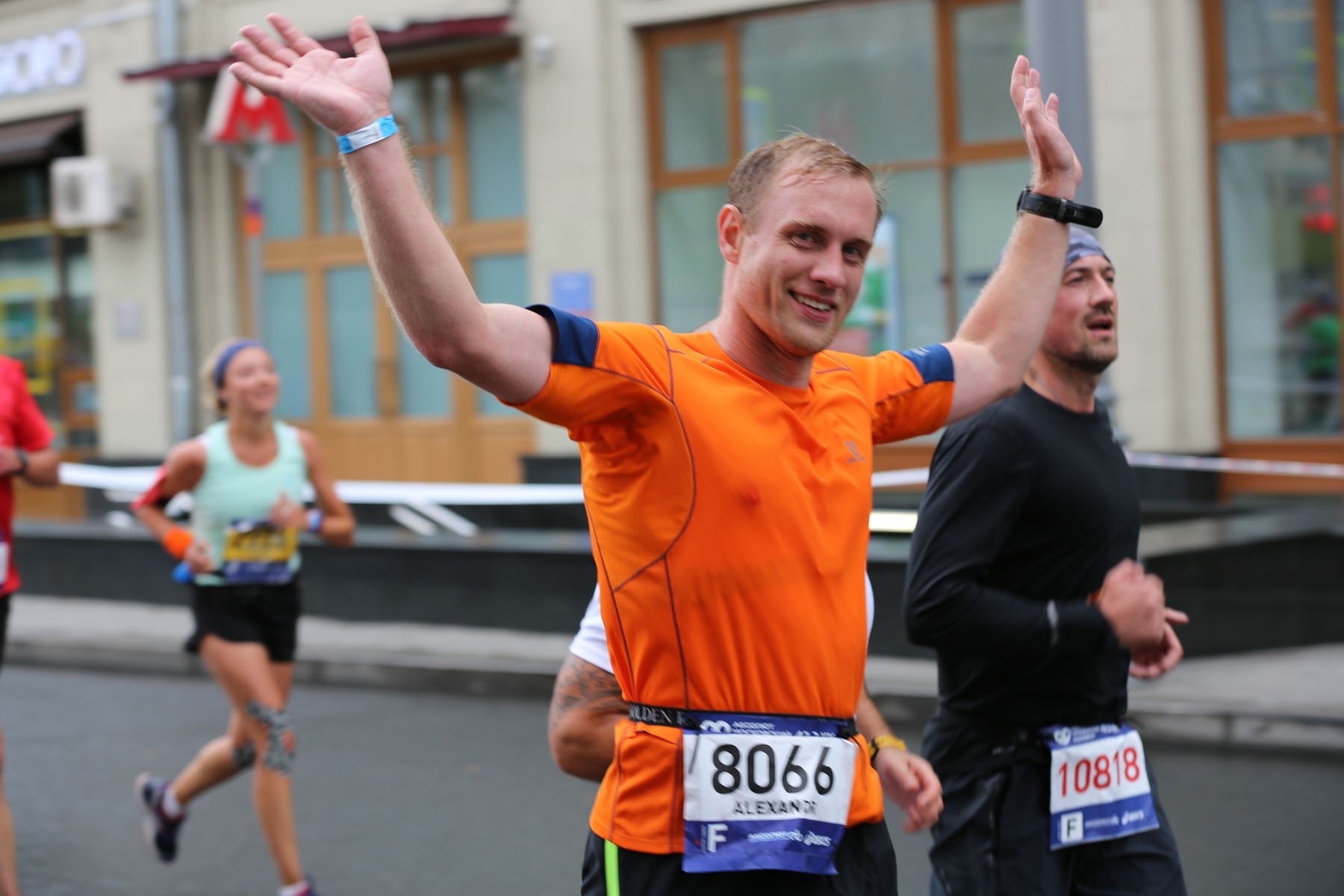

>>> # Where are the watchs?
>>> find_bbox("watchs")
[1014,187,1104,230]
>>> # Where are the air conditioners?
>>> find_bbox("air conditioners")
[51,154,119,231]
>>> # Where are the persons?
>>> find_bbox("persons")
[0,350,62,896]
[549,568,944,833]
[226,11,1086,896]
[901,231,1191,896]
[130,338,356,895]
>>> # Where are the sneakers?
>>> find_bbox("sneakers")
[136,772,187,863]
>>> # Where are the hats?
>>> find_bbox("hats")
[1064,226,1113,266]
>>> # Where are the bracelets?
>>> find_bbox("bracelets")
[15,446,30,476]
[336,113,399,155]
[868,733,906,767]
[163,526,194,560]
[309,507,324,533]
